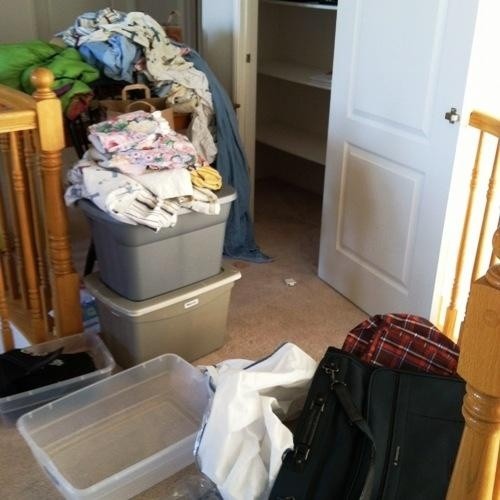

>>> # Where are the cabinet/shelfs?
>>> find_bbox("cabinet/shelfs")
[197,1,489,342]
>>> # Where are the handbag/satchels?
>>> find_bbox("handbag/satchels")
[269,347,467,500]
[99,83,174,133]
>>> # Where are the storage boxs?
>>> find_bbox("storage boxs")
[75,181,244,369]
